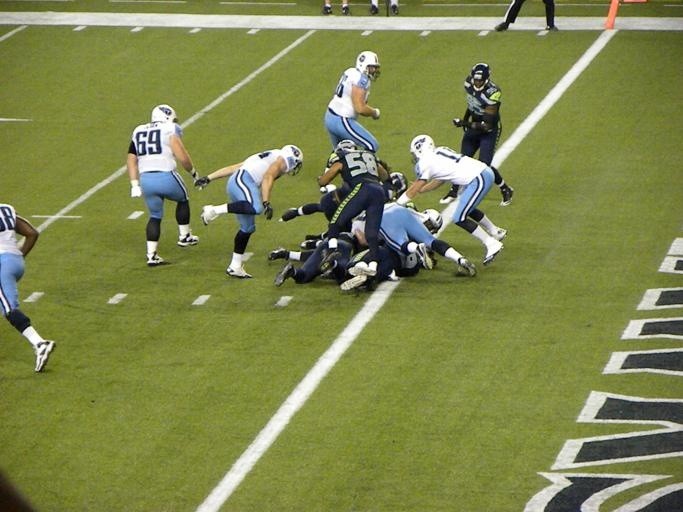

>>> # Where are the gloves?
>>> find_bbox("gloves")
[261,201,273,220]
[194,176,209,192]
[130,186,141,197]
[192,170,198,185]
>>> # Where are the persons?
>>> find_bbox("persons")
[319,0,352,15]
[494,0,559,30]
[267,139,477,291]
[396,134,507,264]
[438,63,514,206]
[0,203,56,372]
[324,50,382,153]
[126,103,199,265]
[194,144,303,278]
[369,0,399,14]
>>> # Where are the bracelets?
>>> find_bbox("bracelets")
[373,107,380,119]
[130,179,138,186]
[189,167,195,175]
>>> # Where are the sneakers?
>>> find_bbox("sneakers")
[200,204,217,226]
[495,22,508,31]
[267,187,513,291]
[321,5,399,16]
[226,265,252,278]
[32,340,55,372]
[144,251,164,265]
[177,231,199,247]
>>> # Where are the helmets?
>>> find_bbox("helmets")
[470,63,489,81]
[150,104,177,123]
[280,145,304,173]
[334,140,355,150]
[408,135,435,162]
[355,51,381,68]
[420,208,443,234]
[389,172,407,193]
